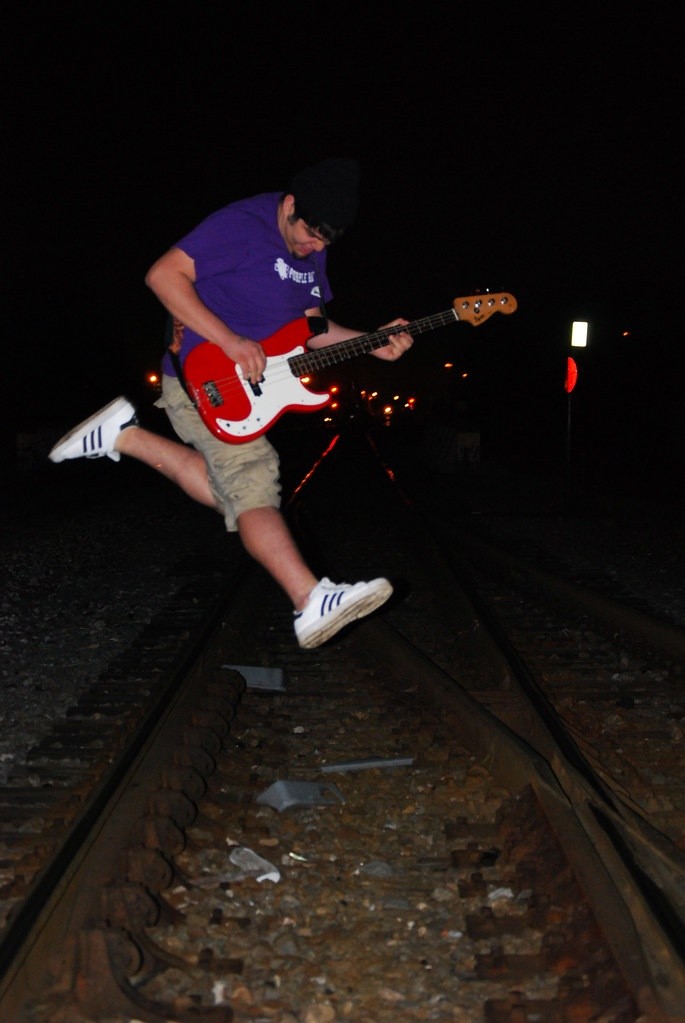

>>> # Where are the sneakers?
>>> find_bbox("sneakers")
[292,576,393,650]
[48,398,138,462]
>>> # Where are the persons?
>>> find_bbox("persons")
[49,176,413,649]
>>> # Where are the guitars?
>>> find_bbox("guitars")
[181,285,521,446]
